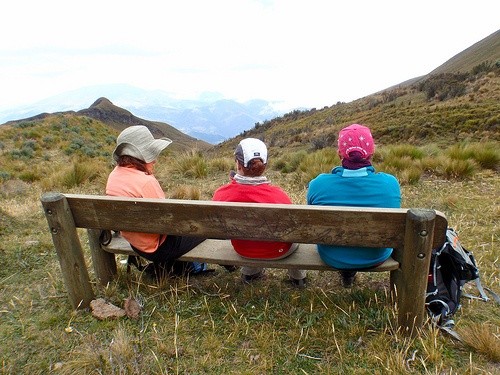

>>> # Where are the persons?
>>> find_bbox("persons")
[104,124,239,276]
[212,137,311,288]
[305,122,403,289]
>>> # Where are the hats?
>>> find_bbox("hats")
[113,124,173,164]
[337,123,375,160]
[234,138,268,168]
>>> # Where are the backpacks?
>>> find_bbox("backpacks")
[424,227,489,341]
[127,254,216,279]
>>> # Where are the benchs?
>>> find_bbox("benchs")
[41,190,450,332]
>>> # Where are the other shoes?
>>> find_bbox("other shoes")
[341,277,354,288]
[240,266,265,280]
[288,269,307,285]
[229,265,240,273]
